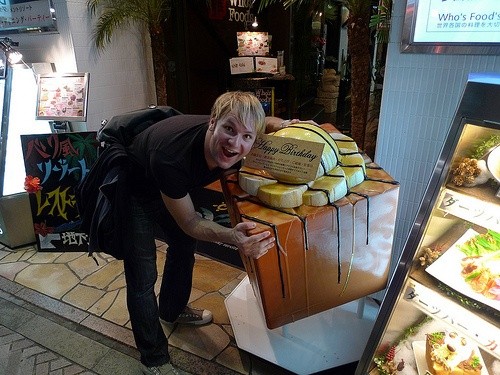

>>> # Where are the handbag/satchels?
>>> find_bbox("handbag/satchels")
[96,104,184,148]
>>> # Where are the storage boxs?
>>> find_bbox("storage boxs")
[316,75,339,112]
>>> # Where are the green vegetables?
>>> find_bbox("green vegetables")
[431,332,480,368]
[457,229,500,281]
[471,133,500,159]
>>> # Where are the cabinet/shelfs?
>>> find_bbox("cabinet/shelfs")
[358,73,500,375]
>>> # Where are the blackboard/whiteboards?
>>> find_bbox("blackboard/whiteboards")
[20,130,99,251]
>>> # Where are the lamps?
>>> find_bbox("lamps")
[0,40,23,65]
[252,13,259,28]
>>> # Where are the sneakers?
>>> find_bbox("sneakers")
[142,362,182,375]
[159,305,213,325]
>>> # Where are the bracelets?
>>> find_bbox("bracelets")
[284,120,290,123]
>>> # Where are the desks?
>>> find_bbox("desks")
[240,73,296,120]
[219,122,399,337]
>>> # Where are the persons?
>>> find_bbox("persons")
[89,92,321,375]
